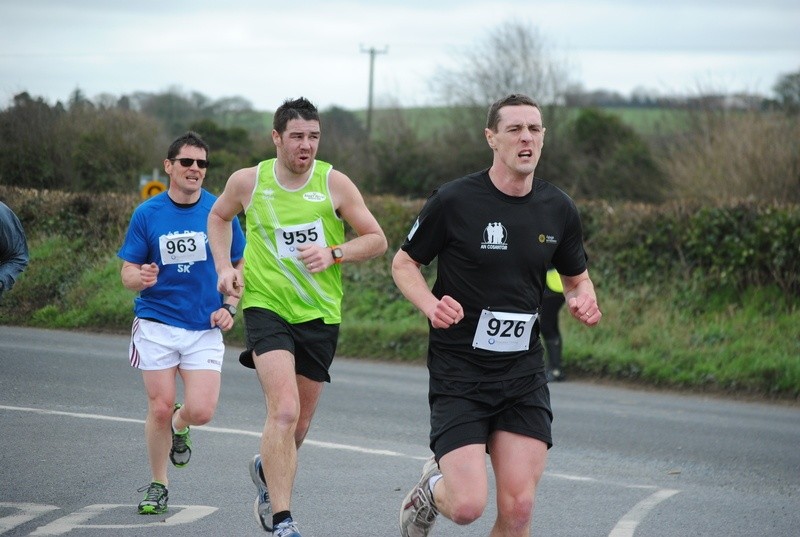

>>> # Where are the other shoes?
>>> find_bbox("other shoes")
[546,370,565,383]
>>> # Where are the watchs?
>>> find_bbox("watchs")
[219,303,237,318]
[329,245,343,264]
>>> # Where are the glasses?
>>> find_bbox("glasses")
[171,158,210,168]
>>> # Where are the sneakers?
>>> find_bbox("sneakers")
[137,480,168,514]
[169,403,192,469]
[400,456,440,537]
[272,518,301,537]
[249,454,272,536]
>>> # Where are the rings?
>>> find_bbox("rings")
[586,312,591,317]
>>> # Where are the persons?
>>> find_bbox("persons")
[117,131,247,514]
[206,95,388,537]
[537,268,565,383]
[0,201,29,296]
[391,93,602,536]
